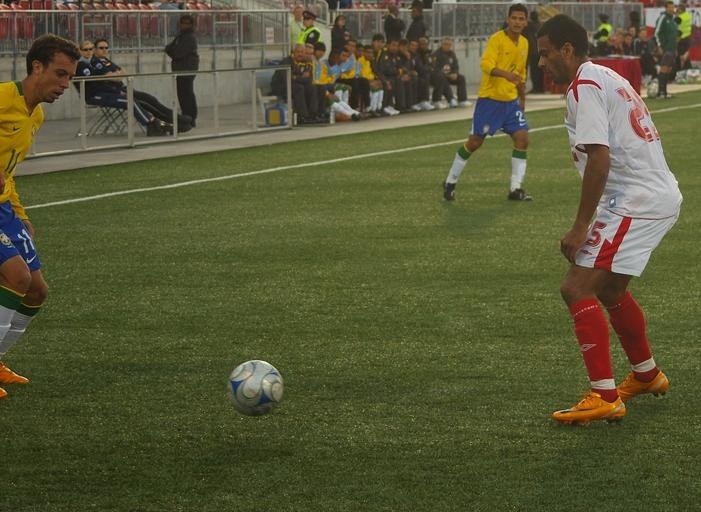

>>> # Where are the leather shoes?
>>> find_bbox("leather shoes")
[301,115,327,124]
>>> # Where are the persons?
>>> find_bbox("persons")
[592,2,692,98]
[537,14,684,425]
[0,32,81,398]
[442,5,533,201]
[165,15,199,127]
[91,40,193,133]
[270,0,473,121]
[72,40,173,134]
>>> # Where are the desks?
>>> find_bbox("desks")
[542,55,641,97]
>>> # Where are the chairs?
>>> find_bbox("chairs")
[0,0,250,52]
[70,75,146,137]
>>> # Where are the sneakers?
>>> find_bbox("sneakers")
[0,388,8,398]
[655,94,672,99]
[350,99,473,120]
[509,188,533,201]
[147,115,195,136]
[616,370,668,401]
[443,181,456,200]
[0,362,30,384]
[552,391,625,426]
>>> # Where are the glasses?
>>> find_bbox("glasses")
[100,47,108,49]
[80,48,92,51]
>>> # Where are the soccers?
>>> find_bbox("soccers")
[226,359,284,416]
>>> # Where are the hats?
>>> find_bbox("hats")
[598,13,608,21]
[302,11,316,20]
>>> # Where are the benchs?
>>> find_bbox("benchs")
[247,69,279,129]
